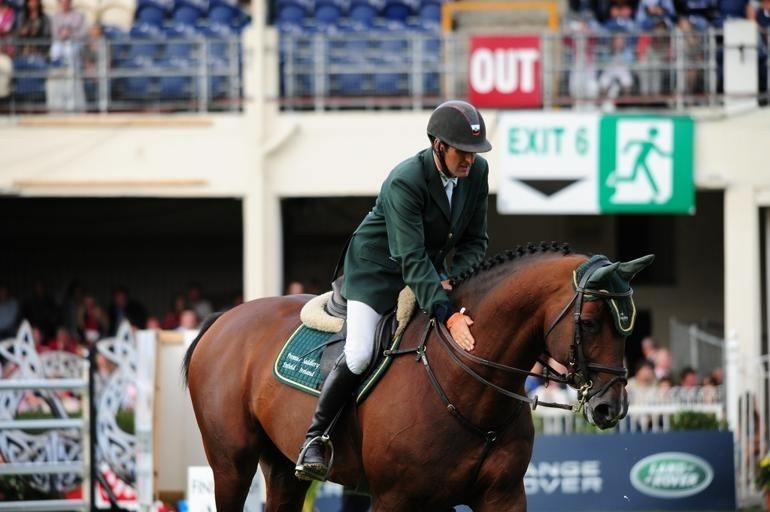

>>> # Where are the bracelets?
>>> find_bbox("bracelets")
[445,306,464,330]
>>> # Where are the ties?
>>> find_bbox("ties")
[445,180,453,208]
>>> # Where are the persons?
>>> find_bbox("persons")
[0,0,110,115]
[520,336,763,482]
[292,99,493,481]
[549,1,770,111]
[1,272,328,414]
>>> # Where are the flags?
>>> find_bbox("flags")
[65,461,178,512]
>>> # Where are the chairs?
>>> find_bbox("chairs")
[14,1,442,97]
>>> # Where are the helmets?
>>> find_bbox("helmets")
[427,100,492,153]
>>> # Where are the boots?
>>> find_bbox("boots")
[295,349,363,482]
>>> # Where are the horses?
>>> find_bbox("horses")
[182,242,656,512]
